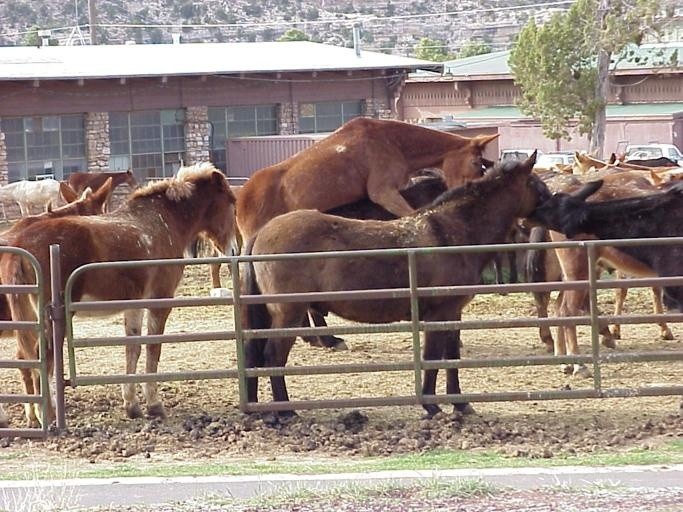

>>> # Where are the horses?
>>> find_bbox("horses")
[498,147,681,355]
[3,167,139,223]
[236,146,555,428]
[295,154,452,351]
[229,117,502,251]
[0,158,237,430]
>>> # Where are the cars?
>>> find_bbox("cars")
[502,149,577,171]
[627,143,683,162]
